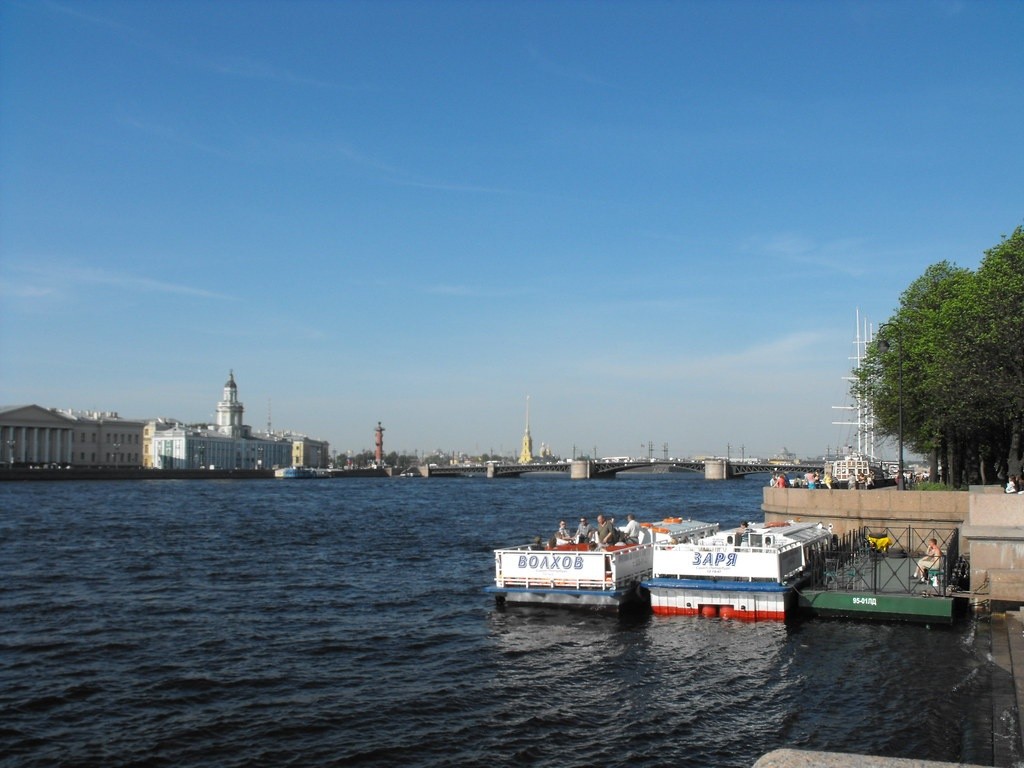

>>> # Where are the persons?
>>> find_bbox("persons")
[596,516,614,545]
[895,470,915,488]
[773,476,786,488]
[739,522,754,552]
[823,472,832,489]
[793,474,803,488]
[770,470,841,490]
[908,539,941,583]
[847,471,875,491]
[1003,474,1024,494]
[533,516,628,551]
[620,513,643,544]
[558,520,573,540]
[575,518,596,544]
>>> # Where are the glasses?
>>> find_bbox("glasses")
[580,520,585,522]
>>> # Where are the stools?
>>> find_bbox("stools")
[927,571,944,586]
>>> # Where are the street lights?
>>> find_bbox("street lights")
[835,446,840,460]
[739,444,746,463]
[826,444,832,463]
[877,324,906,490]
[726,443,732,461]
[8,440,16,469]
[113,443,121,468]
[572,444,578,460]
[198,444,205,469]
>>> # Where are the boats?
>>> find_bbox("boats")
[788,453,886,485]
[485,517,718,614]
[639,520,838,624]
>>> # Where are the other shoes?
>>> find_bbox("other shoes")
[917,580,925,584]
[909,575,918,579]
